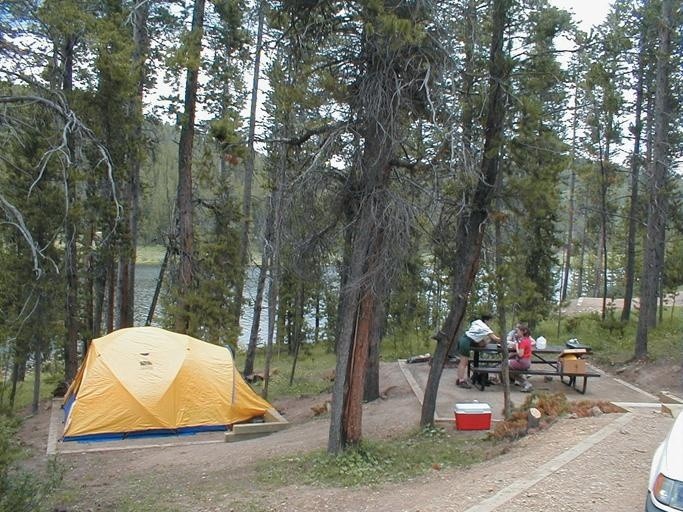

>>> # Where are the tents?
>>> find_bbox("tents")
[55,327,271,443]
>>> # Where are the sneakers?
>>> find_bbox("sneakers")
[515,381,521,385]
[456,379,470,389]
[521,383,533,392]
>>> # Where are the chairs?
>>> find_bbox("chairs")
[457,341,601,394]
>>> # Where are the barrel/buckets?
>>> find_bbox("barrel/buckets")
[535,336,547,349]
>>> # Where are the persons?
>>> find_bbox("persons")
[505,322,535,348]
[507,325,533,393]
[454,312,516,389]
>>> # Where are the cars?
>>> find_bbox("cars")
[643,410,683,512]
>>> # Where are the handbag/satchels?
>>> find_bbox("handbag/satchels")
[472,372,488,386]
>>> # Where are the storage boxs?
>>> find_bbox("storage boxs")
[453,403,492,431]
